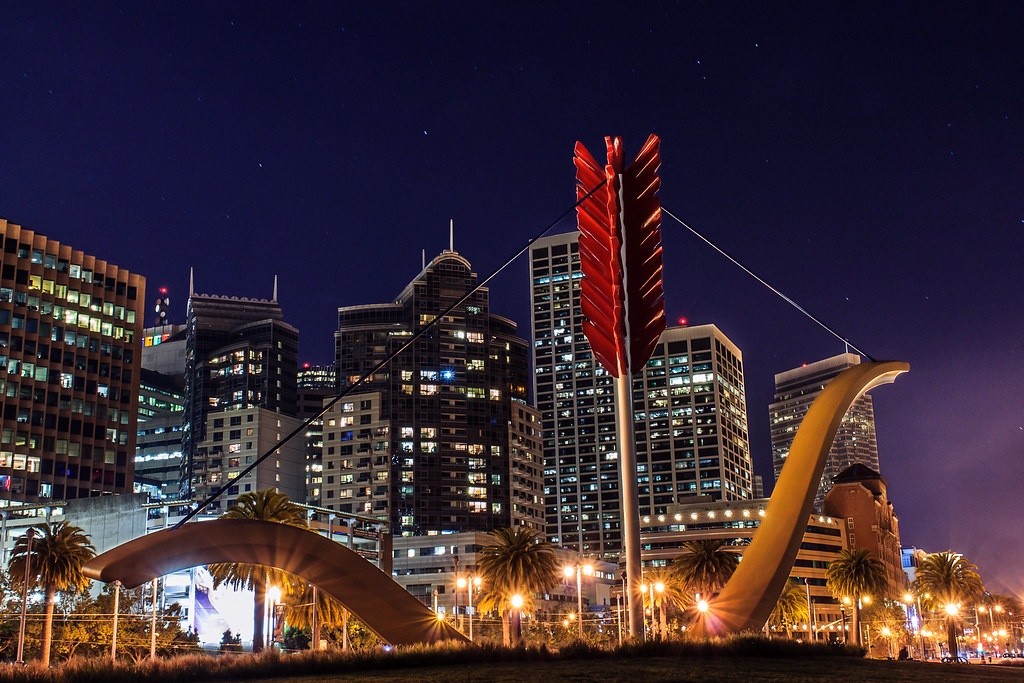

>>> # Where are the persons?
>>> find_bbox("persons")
[899,647,908,661]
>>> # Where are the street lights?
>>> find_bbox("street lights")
[641,582,664,642]
[270,585,281,648]
[904,592,931,659]
[845,597,870,647]
[989,606,1001,629]
[458,575,482,642]
[974,603,985,641]
[563,562,593,638]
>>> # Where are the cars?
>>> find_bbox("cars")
[969,651,977,658]
